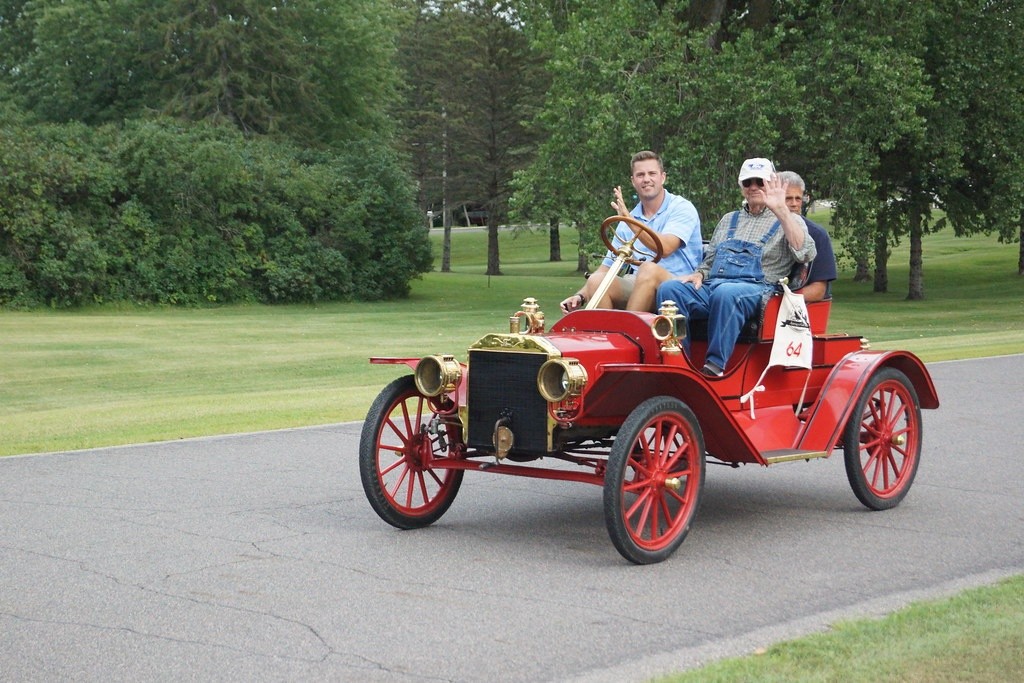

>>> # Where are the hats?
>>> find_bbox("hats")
[737,157,777,191]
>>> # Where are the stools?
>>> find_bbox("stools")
[805,294,833,336]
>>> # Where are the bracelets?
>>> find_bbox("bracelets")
[576,293,585,306]
[698,269,704,280]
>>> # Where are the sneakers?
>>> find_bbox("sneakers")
[703,363,723,376]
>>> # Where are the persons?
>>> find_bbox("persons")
[559,150,702,315]
[655,158,817,376]
[777,170,836,302]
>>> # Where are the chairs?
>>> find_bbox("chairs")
[693,256,813,343]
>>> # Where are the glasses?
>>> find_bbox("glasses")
[742,179,765,188]
